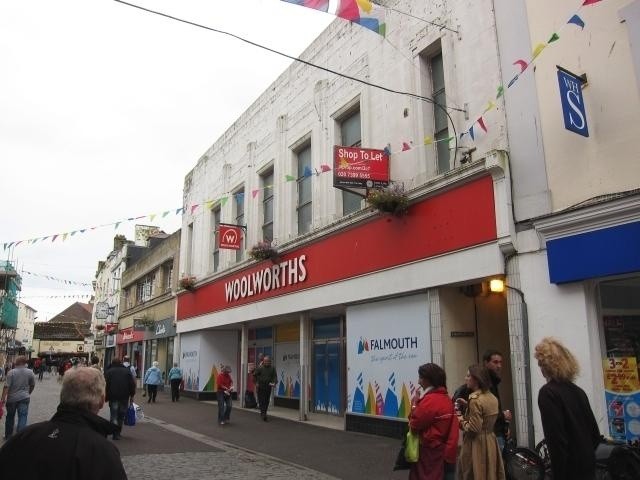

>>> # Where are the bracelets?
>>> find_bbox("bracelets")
[412,406,416,409]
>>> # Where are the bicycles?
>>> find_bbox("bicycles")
[534,431,638,480]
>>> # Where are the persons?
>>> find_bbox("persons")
[250,353,265,409]
[253,356,277,421]
[535,338,599,480]
[479,349,513,454]
[455,364,505,480]
[408,364,460,480]
[217,365,234,425]
[0,352,182,479]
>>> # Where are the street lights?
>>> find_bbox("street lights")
[488,278,536,452]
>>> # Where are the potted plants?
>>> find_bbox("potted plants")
[367,182,411,212]
[248,240,276,263]
[173,276,197,290]
[140,316,154,326]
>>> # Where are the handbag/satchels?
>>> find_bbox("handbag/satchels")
[124,405,135,425]
[404,432,420,463]
